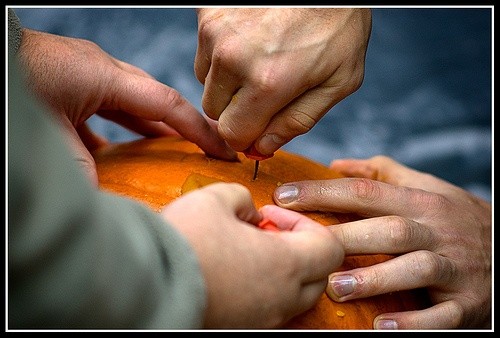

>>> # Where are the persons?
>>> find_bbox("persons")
[192,8,490,330]
[7,9,344,329]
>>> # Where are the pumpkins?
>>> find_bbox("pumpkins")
[87,136,432,329]
